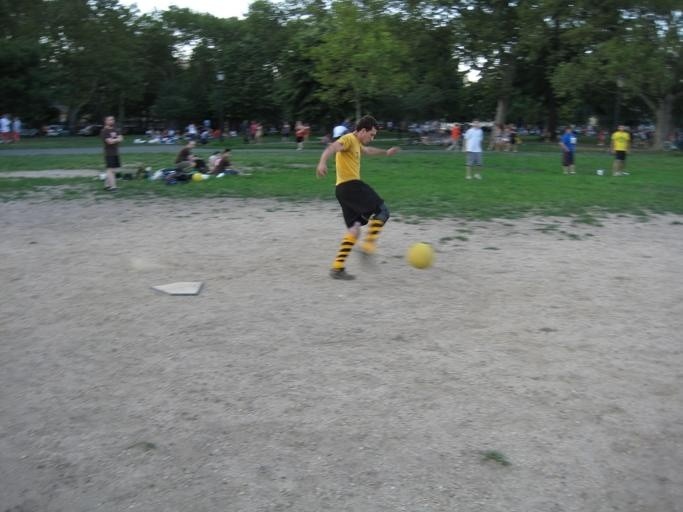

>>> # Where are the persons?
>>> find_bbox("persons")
[313,115,405,285]
[145,116,357,184]
[609,123,631,177]
[557,124,577,174]
[11,116,23,143]
[462,117,485,181]
[0,109,11,144]
[101,113,125,192]
[398,115,682,153]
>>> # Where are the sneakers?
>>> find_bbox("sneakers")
[465,169,632,181]
[328,268,356,281]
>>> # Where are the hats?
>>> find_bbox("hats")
[333,125,348,139]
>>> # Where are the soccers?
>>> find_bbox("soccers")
[192,173,203,182]
[405,243,435,268]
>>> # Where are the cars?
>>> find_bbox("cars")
[264,127,280,136]
[76,124,105,136]
[42,125,72,138]
